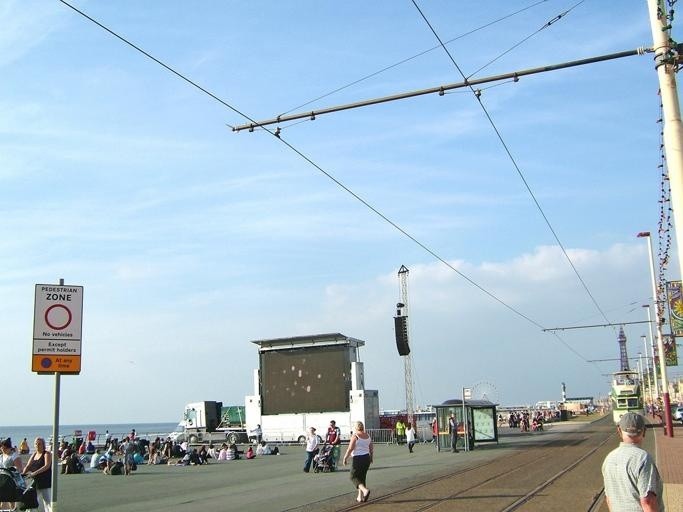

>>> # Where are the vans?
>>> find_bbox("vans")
[166,422,184,444]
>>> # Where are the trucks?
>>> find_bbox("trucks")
[181,390,380,447]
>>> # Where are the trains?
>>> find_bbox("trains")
[608,371,645,428]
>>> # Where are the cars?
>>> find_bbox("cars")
[646,405,683,423]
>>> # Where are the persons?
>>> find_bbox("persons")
[58,442,94,474]
[208,443,256,460]
[598,411,667,511]
[90,429,136,474]
[323,420,341,470]
[0,436,52,512]
[343,421,372,502]
[261,441,280,455]
[303,426,320,472]
[499,411,543,432]
[149,437,208,465]
[397,420,405,445]
[449,413,459,453]
[405,423,416,453]
[250,424,262,444]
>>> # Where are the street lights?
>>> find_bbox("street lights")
[642,304,660,417]
[636,352,647,416]
[640,335,655,418]
[637,230,673,440]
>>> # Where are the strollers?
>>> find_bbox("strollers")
[312,443,336,474]
[0,467,39,512]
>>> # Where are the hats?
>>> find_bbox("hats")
[617,413,644,434]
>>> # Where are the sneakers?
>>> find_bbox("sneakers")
[357,489,370,502]
